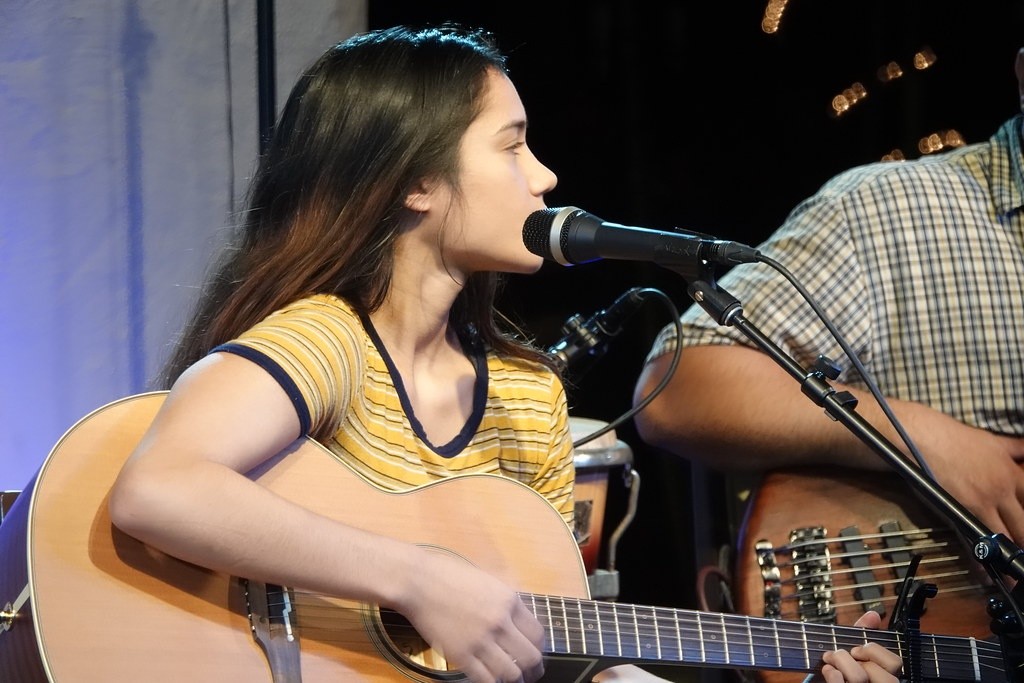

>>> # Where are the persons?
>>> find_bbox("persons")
[110,24,902,683]
[633,47,1023,585]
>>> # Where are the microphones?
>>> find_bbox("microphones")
[521,204,760,271]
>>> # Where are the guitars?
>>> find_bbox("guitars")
[0,381,1023,683]
